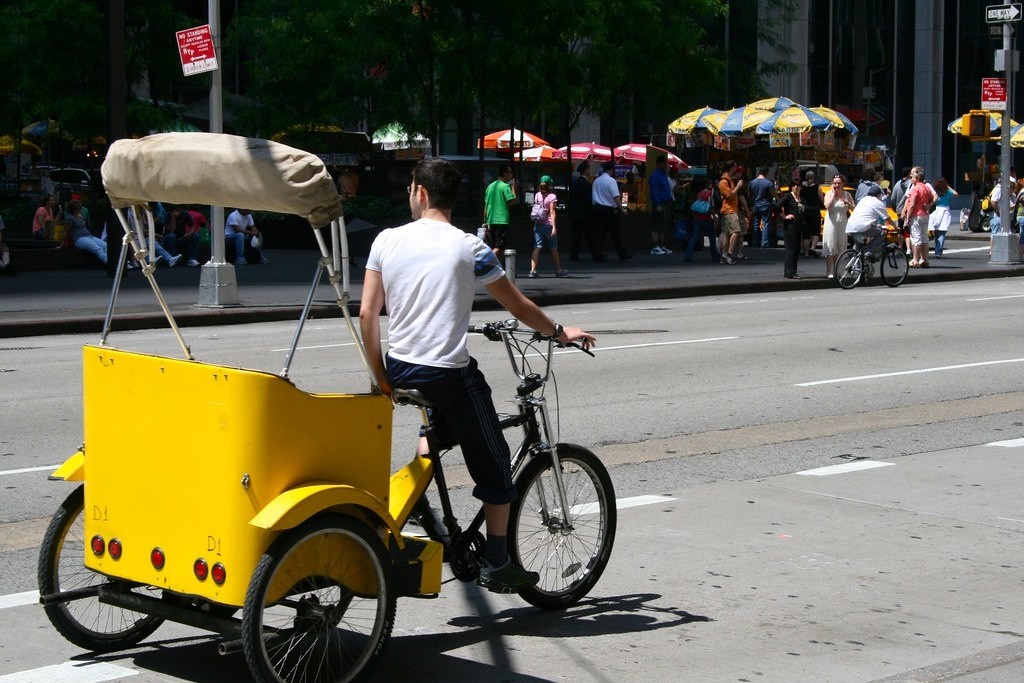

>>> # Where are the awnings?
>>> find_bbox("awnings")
[424,143,511,163]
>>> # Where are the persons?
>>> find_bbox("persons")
[0,215,10,271]
[30,187,274,271]
[355,155,597,591]
[565,160,608,263]
[481,164,518,258]
[619,155,1024,280]
[335,165,360,198]
[527,174,569,279]
[592,160,634,263]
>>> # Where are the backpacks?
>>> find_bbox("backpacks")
[531,194,551,222]
[713,178,731,211]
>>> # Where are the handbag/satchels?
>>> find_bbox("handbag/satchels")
[690,198,711,214]
[250,233,262,248]
[981,199,994,214]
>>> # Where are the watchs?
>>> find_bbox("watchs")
[549,322,564,339]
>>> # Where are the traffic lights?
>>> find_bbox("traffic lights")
[962,110,990,137]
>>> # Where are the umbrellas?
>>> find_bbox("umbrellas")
[370,119,434,153]
[947,108,1019,190]
[471,126,692,172]
[666,94,861,163]
[0,88,345,174]
[996,122,1024,150]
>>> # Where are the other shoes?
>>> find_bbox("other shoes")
[256,259,268,264]
[236,259,247,265]
[906,249,912,257]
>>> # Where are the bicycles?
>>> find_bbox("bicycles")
[833,228,909,288]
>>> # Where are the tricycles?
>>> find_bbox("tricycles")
[38,132,620,683]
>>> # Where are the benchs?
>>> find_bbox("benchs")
[82,343,393,507]
[9,244,237,272]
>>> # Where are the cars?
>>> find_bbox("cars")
[528,186,570,211]
[746,183,906,248]
[47,165,95,195]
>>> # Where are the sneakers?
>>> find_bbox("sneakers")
[720,252,736,264]
[650,247,666,255]
[661,247,673,254]
[187,259,200,267]
[169,254,183,268]
[919,259,929,268]
[475,552,540,590]
[155,255,163,268]
[529,270,541,278]
[904,259,919,268]
[555,268,569,278]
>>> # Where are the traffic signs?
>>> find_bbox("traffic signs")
[988,24,1018,39]
[986,3,1022,23]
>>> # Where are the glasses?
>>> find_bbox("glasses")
[406,185,420,193]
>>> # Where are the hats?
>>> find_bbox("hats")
[576,159,591,171]
[869,186,884,195]
[601,159,616,169]
[541,176,553,183]
